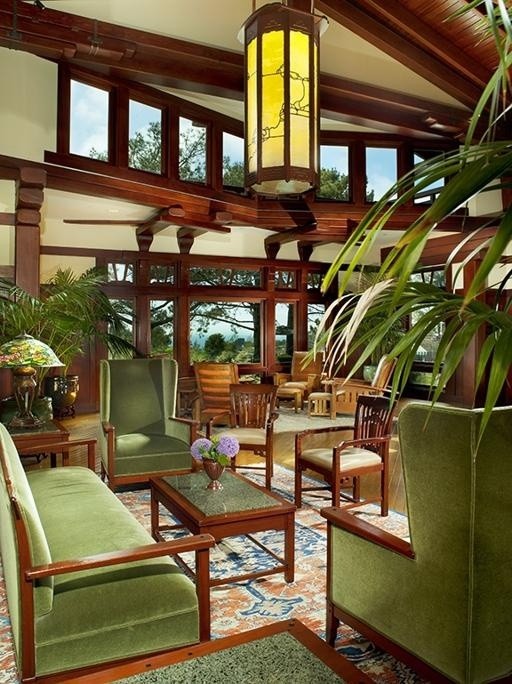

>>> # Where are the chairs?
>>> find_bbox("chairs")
[204,381,280,491]
[90,355,205,493]
[315,398,512,684]
[270,349,324,399]
[176,376,197,419]
[330,352,399,420]
[293,393,398,517]
[188,359,240,429]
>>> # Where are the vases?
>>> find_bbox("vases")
[200,459,226,490]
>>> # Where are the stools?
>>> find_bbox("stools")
[306,390,332,417]
[276,386,300,414]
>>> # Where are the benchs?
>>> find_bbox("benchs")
[1,418,218,683]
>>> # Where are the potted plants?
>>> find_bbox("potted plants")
[39,263,113,407]
[0,272,151,421]
[349,300,409,382]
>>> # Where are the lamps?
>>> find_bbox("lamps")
[1,325,71,431]
[228,1,333,203]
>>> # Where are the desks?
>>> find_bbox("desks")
[1,416,71,468]
[319,376,342,392]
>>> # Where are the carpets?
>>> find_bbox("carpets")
[271,399,402,435]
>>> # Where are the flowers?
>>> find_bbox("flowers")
[187,429,241,469]
